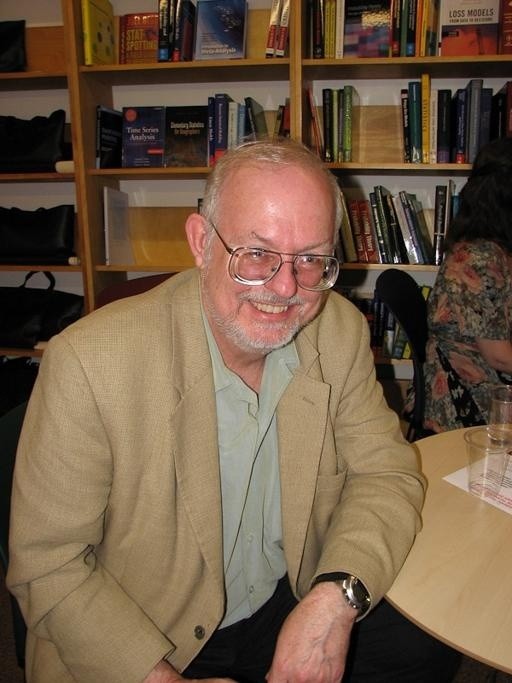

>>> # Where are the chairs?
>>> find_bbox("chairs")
[374,269,438,443]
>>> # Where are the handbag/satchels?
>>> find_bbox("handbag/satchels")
[0,204,75,266]
[0,286,85,351]
[0,109,71,173]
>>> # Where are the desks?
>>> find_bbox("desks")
[382,424,512,683]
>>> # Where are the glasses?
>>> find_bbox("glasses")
[208,219,339,291]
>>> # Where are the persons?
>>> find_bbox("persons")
[405,137,512,440]
[5,135,467,683]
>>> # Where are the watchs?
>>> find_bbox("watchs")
[311,569,371,619]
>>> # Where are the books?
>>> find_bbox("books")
[399,73,512,166]
[94,94,292,168]
[332,179,464,357]
[305,1,511,59]
[81,1,292,65]
[307,86,361,163]
[103,188,134,266]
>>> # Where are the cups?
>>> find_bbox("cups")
[464,427,510,498]
[487,383,512,446]
